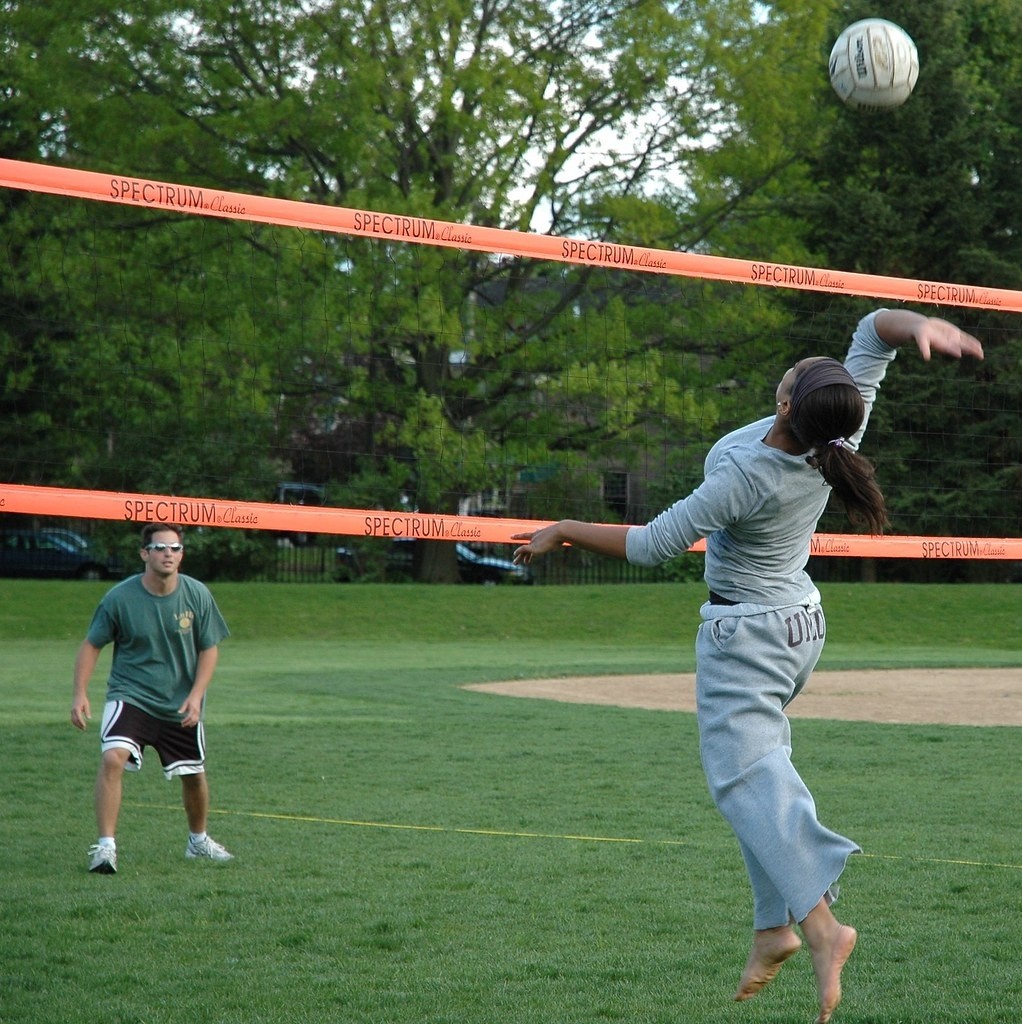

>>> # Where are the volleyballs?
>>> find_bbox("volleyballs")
[828,16,921,110]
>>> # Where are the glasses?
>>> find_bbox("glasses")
[144,543,184,552]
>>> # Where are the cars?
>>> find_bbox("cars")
[267,482,326,546]
[0,525,111,583]
[335,538,535,587]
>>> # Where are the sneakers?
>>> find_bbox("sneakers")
[88,842,118,874]
[186,835,234,861]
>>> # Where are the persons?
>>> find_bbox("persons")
[511,309,983,1024]
[71,522,235,873]
